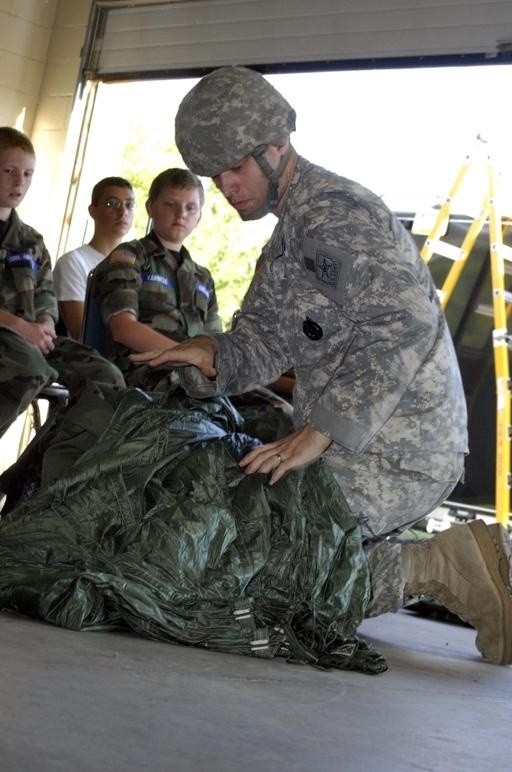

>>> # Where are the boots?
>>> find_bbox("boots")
[402,519,512,665]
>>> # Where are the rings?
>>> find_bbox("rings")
[277,454,283,462]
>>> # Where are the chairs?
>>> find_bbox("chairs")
[79,262,120,357]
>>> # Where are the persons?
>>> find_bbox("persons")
[0,125,127,459]
[93,166,223,357]
[53,176,137,340]
[127,64,509,666]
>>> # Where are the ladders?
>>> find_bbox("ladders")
[418,144,512,538]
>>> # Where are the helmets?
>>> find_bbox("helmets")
[175,67,296,177]
